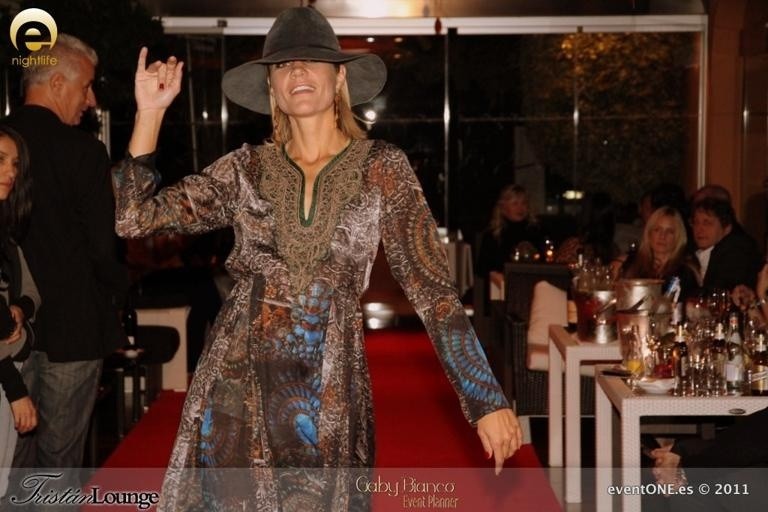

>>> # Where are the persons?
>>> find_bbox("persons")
[126,232,221,372]
[114,8,524,512]
[0,34,128,512]
[0,125,41,499]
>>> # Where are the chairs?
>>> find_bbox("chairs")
[83,280,211,482]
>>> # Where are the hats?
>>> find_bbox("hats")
[219,5,389,116]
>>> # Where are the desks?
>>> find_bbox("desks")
[457,234,767,512]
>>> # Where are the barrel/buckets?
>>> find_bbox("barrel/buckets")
[617,280,667,317]
[618,310,670,374]
[570,279,617,348]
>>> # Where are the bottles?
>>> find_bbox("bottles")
[110,287,139,350]
[632,274,768,396]
[572,246,618,289]
[509,237,556,263]
